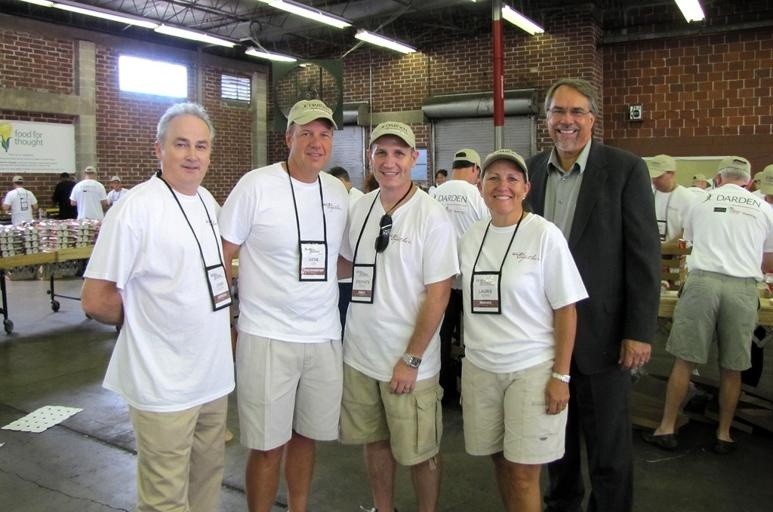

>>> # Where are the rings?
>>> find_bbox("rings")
[640,362,644,366]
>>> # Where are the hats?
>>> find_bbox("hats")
[368,121,416,151]
[480,148,528,183]
[453,148,482,174]
[12,166,120,182]
[647,155,773,196]
[286,100,338,130]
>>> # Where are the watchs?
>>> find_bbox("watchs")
[552,372,571,383]
[402,352,422,368]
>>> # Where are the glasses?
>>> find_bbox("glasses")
[374,214,393,253]
[548,108,592,119]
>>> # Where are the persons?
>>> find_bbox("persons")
[52,166,130,221]
[457,148,590,511]
[429,149,491,407]
[2,175,40,227]
[645,152,773,464]
[80,102,236,512]
[337,121,460,512]
[218,99,350,512]
[521,78,662,512]
[428,170,448,194]
[326,167,365,344]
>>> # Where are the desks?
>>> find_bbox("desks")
[1,245,100,333]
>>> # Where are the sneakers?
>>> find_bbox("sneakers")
[641,431,678,450]
[714,435,737,454]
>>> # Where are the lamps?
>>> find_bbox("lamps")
[502,0,546,36]
[355,28,417,55]
[268,0,354,30]
